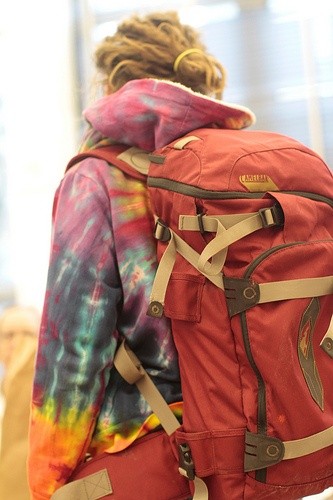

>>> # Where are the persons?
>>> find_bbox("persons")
[28,11,255,500]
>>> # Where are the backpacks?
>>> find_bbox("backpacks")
[52,127,331,500]
